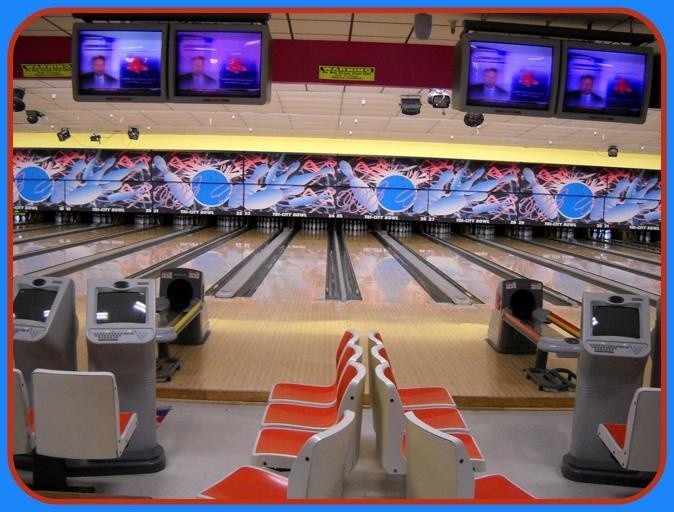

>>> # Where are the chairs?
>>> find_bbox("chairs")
[30,369,137,462]
[599,387,663,472]
[197,410,355,501]
[252,329,486,475]
[11,369,65,491]
[403,409,535,502]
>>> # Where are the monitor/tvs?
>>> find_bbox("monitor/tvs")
[168,22,271,105]
[591,305,640,340]
[555,39,654,124]
[70,22,168,104]
[97,292,145,324]
[452,32,560,119]
[13,288,57,322]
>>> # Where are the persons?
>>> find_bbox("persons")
[469,68,508,98]
[566,74,603,106]
[179,56,217,89]
[79,55,118,88]
